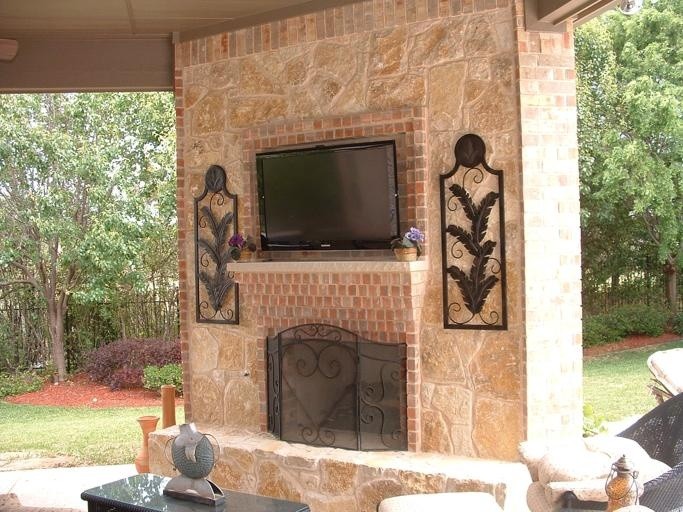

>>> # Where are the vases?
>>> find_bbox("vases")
[135,416,160,473]
[160,385,177,429]
[236,251,252,263]
[394,247,417,262]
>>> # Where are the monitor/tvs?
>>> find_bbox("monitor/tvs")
[257,140,400,250]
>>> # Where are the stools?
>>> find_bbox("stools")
[378,491,502,512]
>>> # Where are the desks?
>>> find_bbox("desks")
[82,473,312,512]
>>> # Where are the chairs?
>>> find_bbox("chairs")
[518,347,683,512]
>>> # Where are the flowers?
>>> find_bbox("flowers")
[227,234,256,261]
[390,226,425,257]
